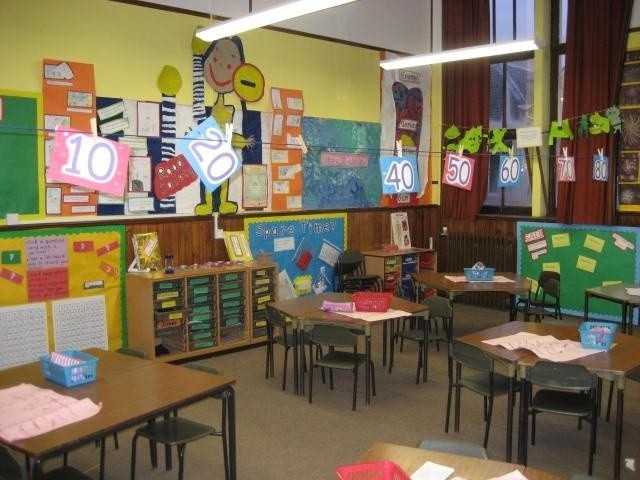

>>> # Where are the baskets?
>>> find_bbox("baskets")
[40,349,99,388]
[464,268,496,280]
[337,460,408,480]
[352,291,392,312]
[578,322,617,350]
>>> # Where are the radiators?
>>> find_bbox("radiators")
[435,229,514,305]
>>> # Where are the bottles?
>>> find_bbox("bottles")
[164,254,175,274]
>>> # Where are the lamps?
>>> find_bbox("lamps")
[195,0,352,49]
[376,0,542,72]
